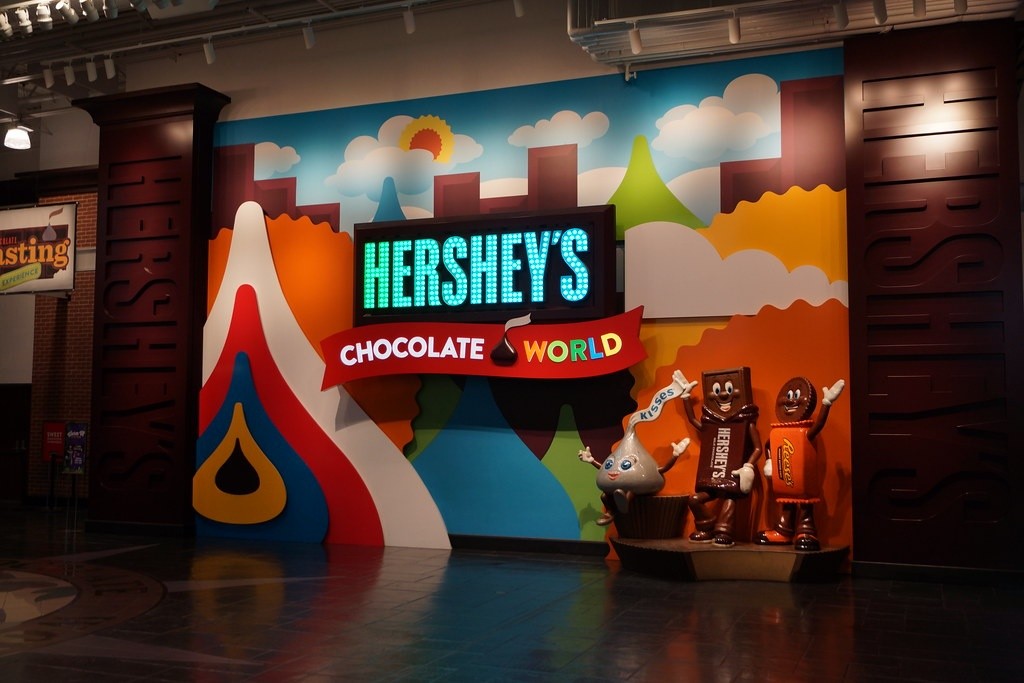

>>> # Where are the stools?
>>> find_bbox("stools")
[600,494,690,540]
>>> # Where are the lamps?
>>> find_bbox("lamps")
[0,13,13,38]
[728,9,741,44]
[85,57,97,83]
[33,2,52,32]
[43,63,55,89]
[4,9,34,33]
[400,4,417,35]
[625,20,643,54]
[64,60,76,86]
[513,0,526,17]
[56,0,184,24]
[954,0,967,14]
[104,52,116,80]
[872,1,889,26]
[203,35,216,64]
[912,0,926,18]
[833,1,849,28]
[301,18,316,50]
